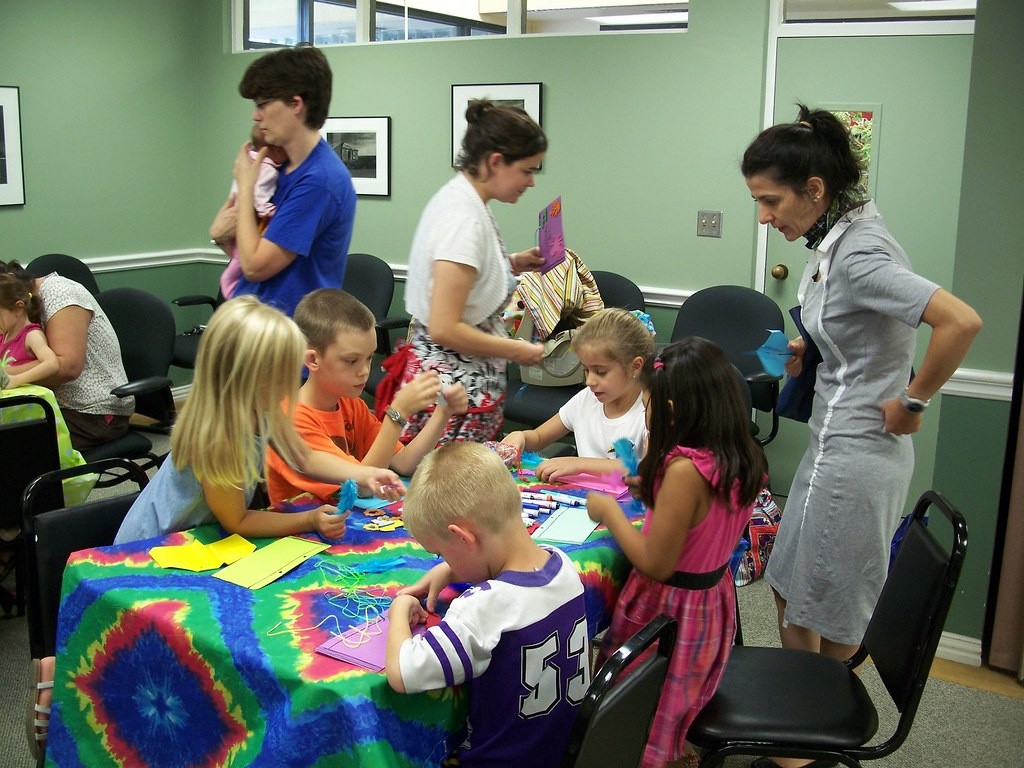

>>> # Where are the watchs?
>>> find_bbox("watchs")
[899,386,931,414]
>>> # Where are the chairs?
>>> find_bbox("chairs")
[557,612,679,768]
[0,394,65,618]
[26,252,788,487]
[21,455,152,659]
[683,487,969,768]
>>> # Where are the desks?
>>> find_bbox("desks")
[42,450,640,768]
[43,445,652,768]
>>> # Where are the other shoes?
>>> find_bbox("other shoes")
[751,756,837,768]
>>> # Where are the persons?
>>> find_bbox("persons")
[262,287,470,509]
[23,293,410,760]
[493,307,656,485]
[586,337,771,768]
[206,42,358,511]
[740,99,983,768]
[384,441,591,768]
[389,98,548,449]
[0,259,135,480]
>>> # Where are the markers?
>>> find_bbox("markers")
[522,503,539,510]
[522,499,560,510]
[539,508,551,514]
[540,489,587,505]
[521,494,534,499]
[520,491,552,501]
[552,495,579,506]
[521,512,533,519]
[523,508,540,517]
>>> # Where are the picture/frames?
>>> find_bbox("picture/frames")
[819,102,881,205]
[450,81,543,171]
[317,116,392,196]
[0,85,26,207]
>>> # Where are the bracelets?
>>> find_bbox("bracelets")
[383,404,410,430]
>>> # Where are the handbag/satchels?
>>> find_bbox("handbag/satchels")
[514,307,586,386]
